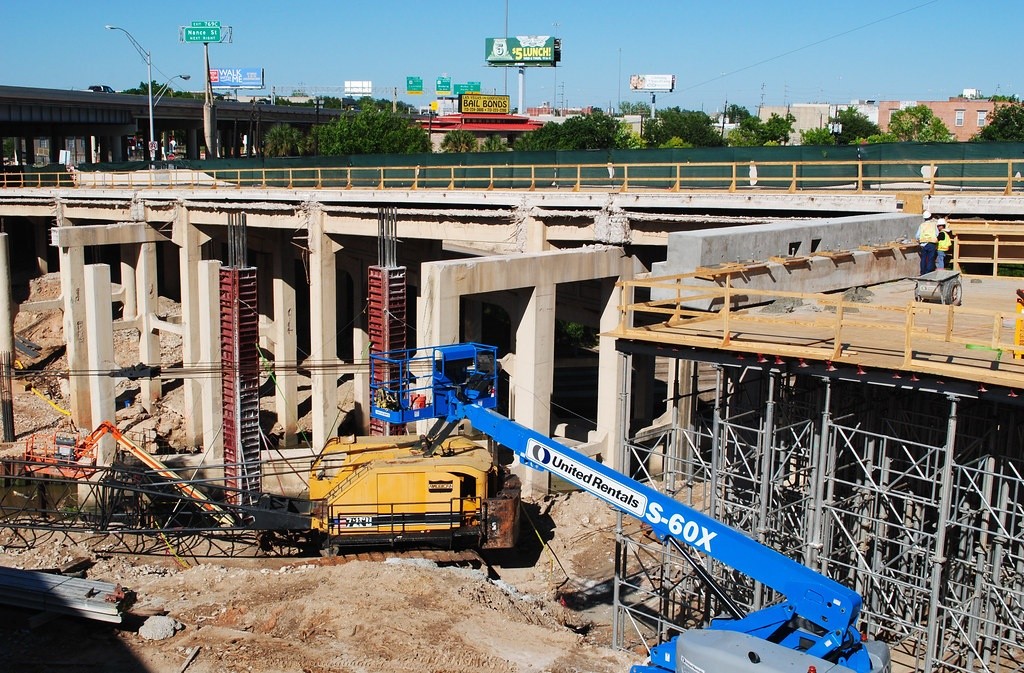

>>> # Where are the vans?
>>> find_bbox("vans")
[423,112,431,116]
[89,86,115,93]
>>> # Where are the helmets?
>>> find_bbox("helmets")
[936,218,947,228]
[922,211,931,219]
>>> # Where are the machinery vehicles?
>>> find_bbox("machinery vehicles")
[370,341,893,673]
[23,432,523,579]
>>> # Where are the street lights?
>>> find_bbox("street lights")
[107,24,191,168]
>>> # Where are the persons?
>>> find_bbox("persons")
[938,218,951,268]
[915,211,940,276]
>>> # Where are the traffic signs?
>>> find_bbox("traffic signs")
[407,78,423,95]
[185,22,221,42]
[454,82,481,94]
[436,77,451,95]
